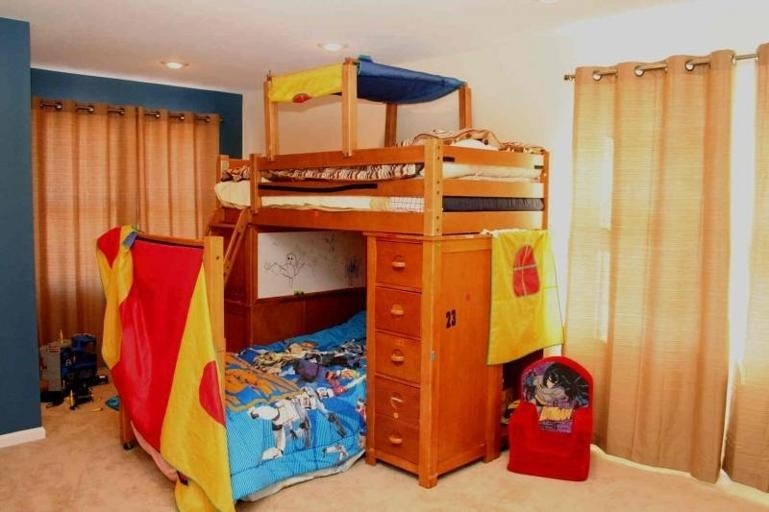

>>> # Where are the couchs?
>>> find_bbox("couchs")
[507,355,596,485]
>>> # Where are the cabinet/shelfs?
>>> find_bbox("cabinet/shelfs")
[361,233,507,489]
[206,225,366,355]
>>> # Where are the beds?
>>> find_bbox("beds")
[213,144,550,234]
[103,227,368,506]
